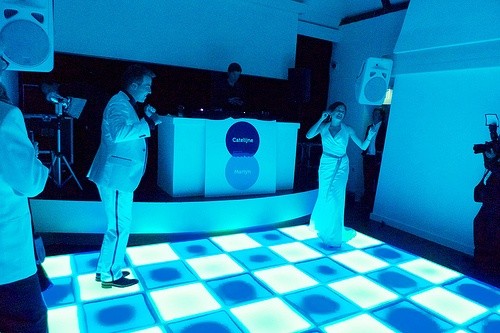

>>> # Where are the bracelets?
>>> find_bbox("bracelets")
[366,138,371,142]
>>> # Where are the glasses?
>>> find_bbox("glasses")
[0,55,9,70]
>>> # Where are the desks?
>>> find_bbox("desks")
[158,116,301,200]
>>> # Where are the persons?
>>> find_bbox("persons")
[473,122,500,277]
[210,62,253,116]
[87,63,163,288]
[0,83,53,333]
[306,102,376,249]
[359,109,387,223]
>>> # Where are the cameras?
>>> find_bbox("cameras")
[473,114,500,160]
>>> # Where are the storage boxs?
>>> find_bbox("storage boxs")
[24,114,73,164]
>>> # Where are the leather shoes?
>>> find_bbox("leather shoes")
[95,271,130,282]
[102,277,139,288]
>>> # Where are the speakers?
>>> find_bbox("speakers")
[355,57,393,106]
[0,0,54,72]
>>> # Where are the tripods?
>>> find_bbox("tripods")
[48,103,84,191]
